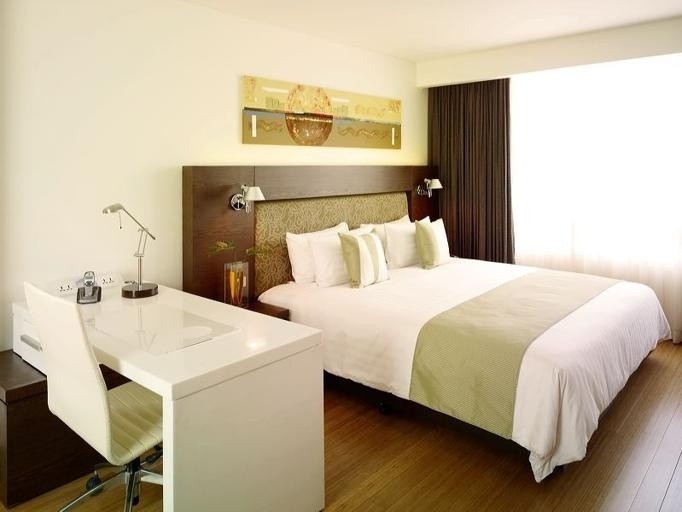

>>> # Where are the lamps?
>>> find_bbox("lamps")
[101,203,158,298]
[231,185,265,212]
[417,178,443,198]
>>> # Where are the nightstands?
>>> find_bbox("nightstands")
[211,298,289,321]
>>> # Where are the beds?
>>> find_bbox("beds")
[183,165,671,483]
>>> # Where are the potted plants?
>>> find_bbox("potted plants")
[207,240,263,308]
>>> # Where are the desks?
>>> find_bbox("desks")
[12,283,325,512]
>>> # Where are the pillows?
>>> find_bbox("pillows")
[384,216,430,269]
[360,214,412,252]
[415,218,451,269]
[286,222,350,285]
[310,224,375,288]
[338,227,390,288]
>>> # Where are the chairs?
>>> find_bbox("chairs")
[22,281,162,512]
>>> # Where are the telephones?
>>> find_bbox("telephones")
[77,271,101,304]
